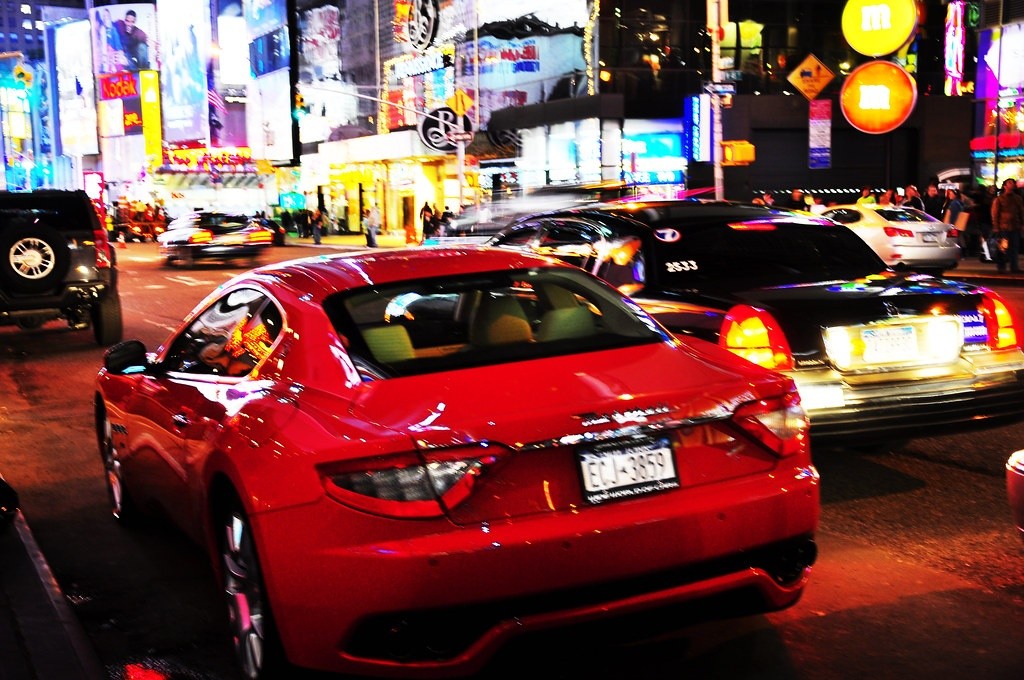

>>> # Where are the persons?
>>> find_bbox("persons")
[926,184,998,259]
[991,177,1024,273]
[144,202,155,220]
[856,187,898,206]
[255,210,266,218]
[790,189,838,213]
[751,191,777,205]
[361,208,379,248]
[899,185,925,212]
[419,201,455,242]
[372,203,382,228]
[95,8,150,73]
[280,209,328,245]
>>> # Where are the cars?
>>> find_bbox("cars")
[481,200,1024,443]
[819,203,961,276]
[158,210,271,267]
[95,244,821,680]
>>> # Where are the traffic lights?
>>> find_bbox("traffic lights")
[720,140,756,165]
[295,93,306,120]
[16,70,25,90]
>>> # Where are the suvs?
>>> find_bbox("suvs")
[0,188,123,347]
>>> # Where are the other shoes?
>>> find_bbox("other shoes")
[997,268,1009,274]
[1011,268,1024,275]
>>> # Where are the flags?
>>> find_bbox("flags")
[209,85,229,115]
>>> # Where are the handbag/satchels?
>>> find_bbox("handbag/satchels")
[980,230,1008,264]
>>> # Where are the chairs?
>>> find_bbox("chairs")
[361,298,599,364]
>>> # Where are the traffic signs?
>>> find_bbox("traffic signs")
[449,134,474,142]
